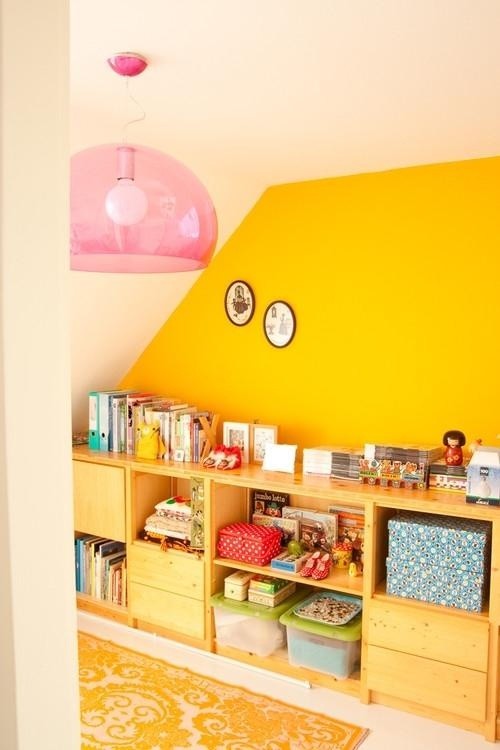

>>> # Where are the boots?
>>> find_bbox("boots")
[202,444,226,467]
[217,446,241,470]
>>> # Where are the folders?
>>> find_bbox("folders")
[88,389,140,451]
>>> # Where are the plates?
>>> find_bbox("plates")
[293,592,363,626]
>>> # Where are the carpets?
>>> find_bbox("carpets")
[76,629,372,750]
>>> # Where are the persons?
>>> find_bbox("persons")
[442,430,466,466]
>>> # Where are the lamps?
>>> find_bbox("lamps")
[71,50,217,274]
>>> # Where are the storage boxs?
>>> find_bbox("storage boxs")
[385,511,491,612]
[211,587,362,683]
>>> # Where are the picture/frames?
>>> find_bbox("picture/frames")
[221,422,278,466]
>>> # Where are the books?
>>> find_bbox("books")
[75,531,129,605]
[302,440,468,493]
[73,430,89,445]
[110,392,214,463]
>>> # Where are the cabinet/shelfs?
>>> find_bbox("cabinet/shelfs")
[71,442,500,744]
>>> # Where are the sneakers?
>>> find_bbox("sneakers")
[313,553,333,580]
[300,551,323,577]
